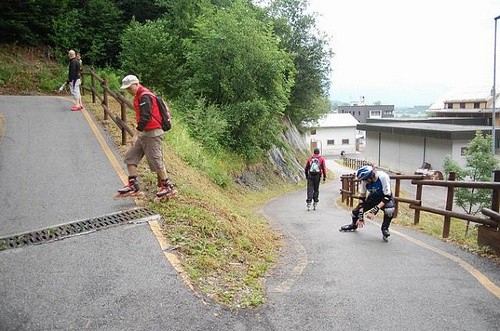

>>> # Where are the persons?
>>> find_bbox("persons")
[305,147,327,211]
[113,74,178,201]
[339,165,395,243]
[66,50,83,111]
[76,52,83,71]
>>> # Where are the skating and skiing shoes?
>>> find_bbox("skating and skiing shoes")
[306,202,311,211]
[113,175,145,201]
[381,225,391,242]
[153,177,178,204]
[313,202,317,210]
[339,222,358,232]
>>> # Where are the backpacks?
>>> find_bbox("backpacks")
[140,92,171,131]
[309,157,320,175]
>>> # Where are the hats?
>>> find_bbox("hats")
[69,50,75,59]
[120,74,139,90]
[314,148,319,152]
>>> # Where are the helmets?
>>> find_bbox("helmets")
[356,165,375,181]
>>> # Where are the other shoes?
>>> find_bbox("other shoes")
[72,105,84,109]
[71,106,81,110]
